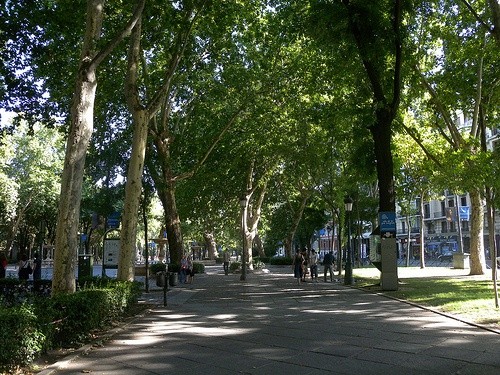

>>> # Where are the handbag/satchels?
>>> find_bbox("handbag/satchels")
[300,254,303,263]
[181,264,187,270]
[27,262,33,274]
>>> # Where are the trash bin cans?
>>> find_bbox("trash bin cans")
[156,271,165,287]
[170,272,178,285]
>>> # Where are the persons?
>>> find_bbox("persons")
[180,254,194,283]
[33,253,41,281]
[309,249,319,283]
[293,248,308,286]
[323,250,335,282]
[18,254,33,281]
[0,253,8,280]
[222,248,230,275]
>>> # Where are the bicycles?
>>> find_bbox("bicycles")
[397,249,451,267]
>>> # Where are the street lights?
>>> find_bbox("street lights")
[239,193,248,281]
[343,193,353,285]
